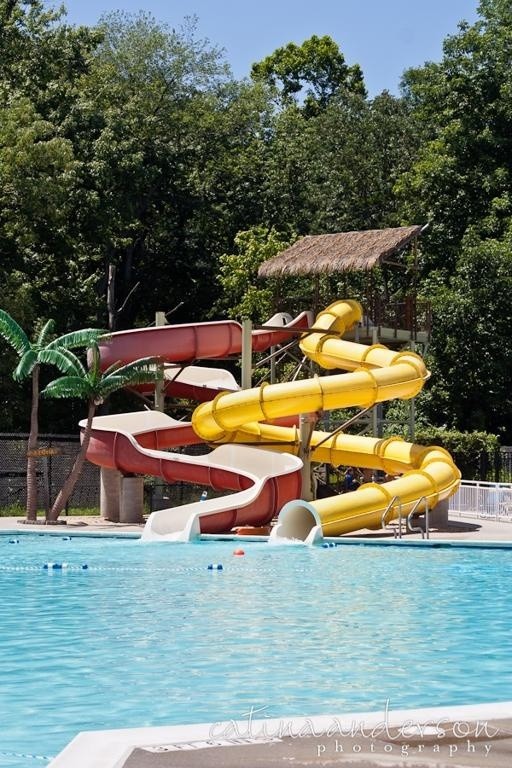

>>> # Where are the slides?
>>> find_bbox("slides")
[80,310,315,542]
